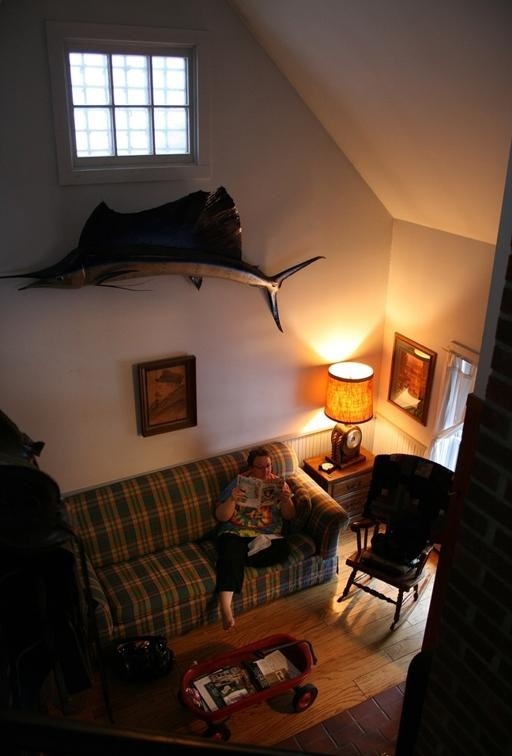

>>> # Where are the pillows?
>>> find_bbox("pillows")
[286,476,311,536]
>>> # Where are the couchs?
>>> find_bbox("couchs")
[57,441,350,646]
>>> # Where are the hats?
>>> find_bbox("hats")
[155,370,184,384]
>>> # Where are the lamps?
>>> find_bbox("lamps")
[324,361,375,470]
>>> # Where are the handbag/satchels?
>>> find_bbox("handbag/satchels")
[109,636,175,684]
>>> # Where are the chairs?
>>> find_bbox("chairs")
[337,454,456,631]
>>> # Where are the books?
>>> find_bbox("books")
[194,649,303,712]
[234,473,283,509]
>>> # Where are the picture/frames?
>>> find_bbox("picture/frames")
[137,354,197,438]
[386,331,438,427]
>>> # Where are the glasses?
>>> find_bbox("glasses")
[254,462,272,470]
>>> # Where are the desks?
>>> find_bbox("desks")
[303,447,375,524]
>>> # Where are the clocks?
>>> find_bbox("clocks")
[345,429,362,449]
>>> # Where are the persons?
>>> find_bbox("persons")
[214,447,296,632]
